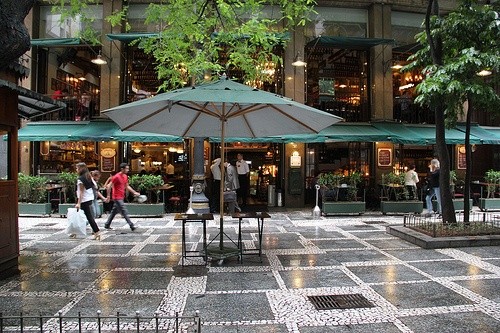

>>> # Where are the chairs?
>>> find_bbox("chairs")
[168,185,192,212]
[453,184,500,207]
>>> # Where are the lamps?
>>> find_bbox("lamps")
[384,55,403,77]
[291,51,307,74]
[338,79,348,89]
[134,147,142,154]
[24,145,28,153]
[75,72,86,82]
[91,49,112,66]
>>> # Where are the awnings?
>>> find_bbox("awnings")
[4,119,500,144]
[306,35,431,74]
[107,30,291,63]
[29,37,102,59]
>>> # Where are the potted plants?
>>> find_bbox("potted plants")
[122,174,164,219]
[381,170,424,214]
[478,169,500,212]
[56,170,104,219]
[432,170,474,213]
[18,172,52,217]
[317,167,367,216]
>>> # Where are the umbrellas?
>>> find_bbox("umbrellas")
[98,73,344,262]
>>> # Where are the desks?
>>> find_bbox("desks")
[333,185,358,201]
[471,181,499,198]
[383,183,404,200]
[149,184,175,213]
[232,211,271,263]
[174,214,214,267]
[32,185,63,212]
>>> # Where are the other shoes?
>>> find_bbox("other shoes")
[421,210,434,214]
[437,215,442,219]
[105,226,113,229]
[132,227,137,231]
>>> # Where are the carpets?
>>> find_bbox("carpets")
[237,204,269,212]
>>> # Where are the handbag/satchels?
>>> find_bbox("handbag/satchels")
[65,208,87,235]
[424,178,433,195]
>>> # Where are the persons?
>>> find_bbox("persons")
[210,157,222,212]
[223,160,241,213]
[141,160,175,180]
[405,164,420,200]
[423,159,442,219]
[233,153,250,210]
[89,169,113,214]
[104,163,141,231]
[69,163,108,240]
[257,168,263,181]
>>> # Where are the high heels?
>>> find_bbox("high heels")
[68,234,77,239]
[91,231,102,241]
